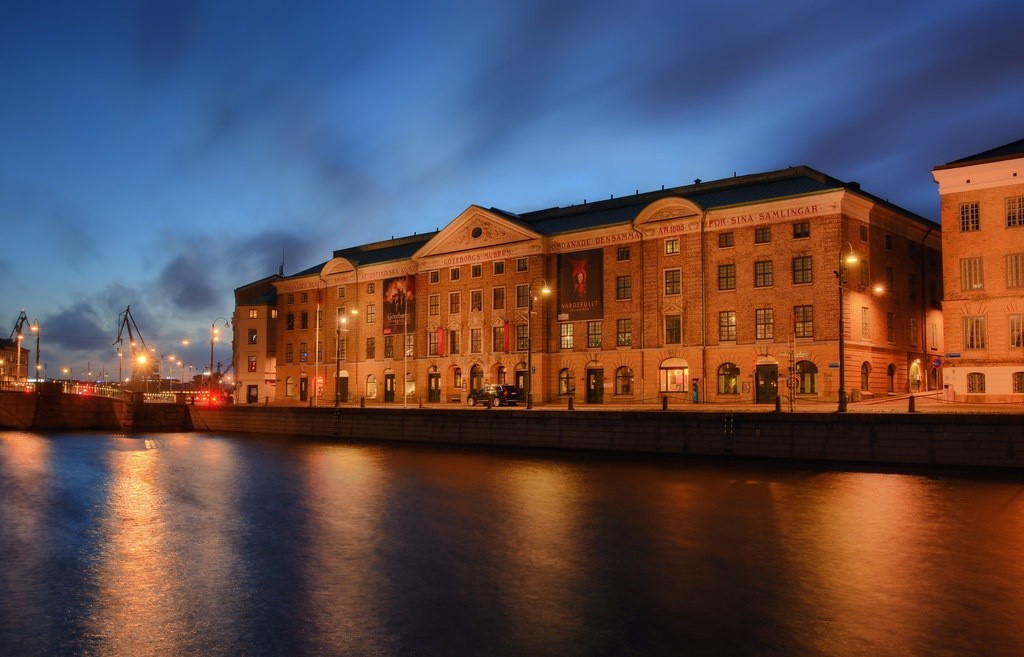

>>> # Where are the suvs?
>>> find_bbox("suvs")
[467,384,519,407]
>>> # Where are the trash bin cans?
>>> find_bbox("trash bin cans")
[943,383,954,404]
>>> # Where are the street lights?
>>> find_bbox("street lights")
[209,317,230,405]
[31,319,40,382]
[838,240,860,413]
[17,332,23,382]
[120,341,135,390]
[140,344,155,392]
[335,300,359,406]
[527,277,550,409]
[158,352,192,393]
